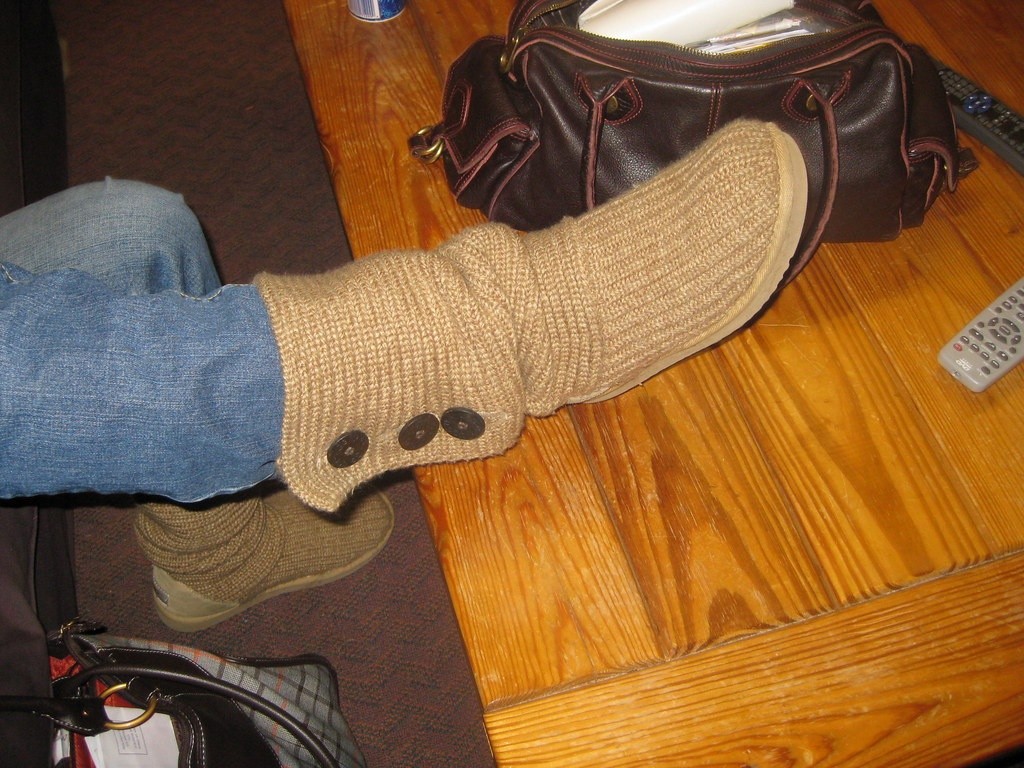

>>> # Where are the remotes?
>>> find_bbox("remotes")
[939,278,1024,394]
[931,60,1024,175]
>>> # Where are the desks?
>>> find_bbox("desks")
[286,1,1024,768]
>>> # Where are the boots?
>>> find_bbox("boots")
[117,488,395,631]
[254,115,808,513]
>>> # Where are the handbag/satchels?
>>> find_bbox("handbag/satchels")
[408,0,979,297]
[0,616,367,768]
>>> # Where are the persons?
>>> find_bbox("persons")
[0,120,808,633]
